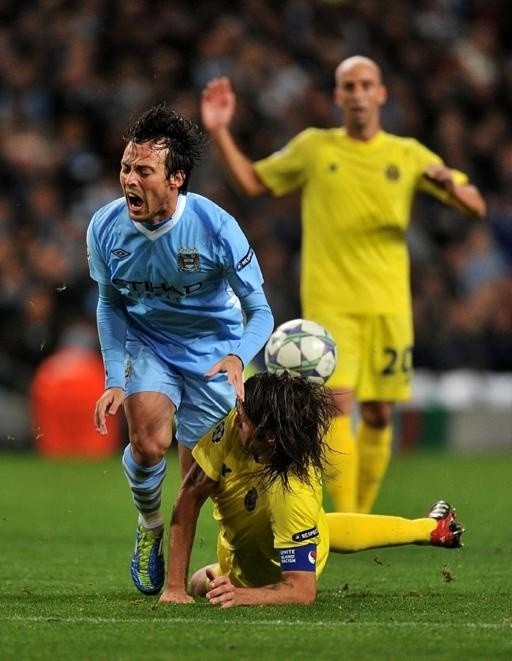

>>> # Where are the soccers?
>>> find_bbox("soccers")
[265,319,337,386]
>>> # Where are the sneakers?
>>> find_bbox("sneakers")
[130,522,165,596]
[428,500,466,549]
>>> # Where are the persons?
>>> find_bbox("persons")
[200,55,487,514]
[86,106,275,595]
[158,372,465,609]
